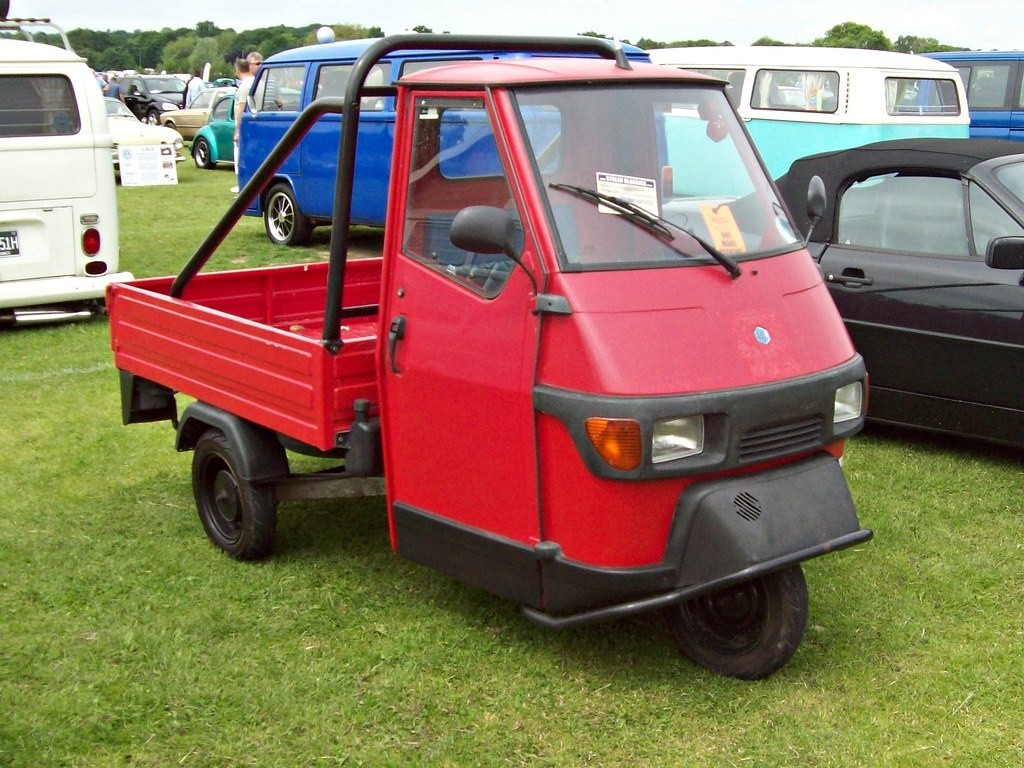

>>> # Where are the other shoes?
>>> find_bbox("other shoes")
[231,185,239,192]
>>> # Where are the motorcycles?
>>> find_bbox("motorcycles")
[106,36,879,680]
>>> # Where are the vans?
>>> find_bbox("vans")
[643,44,972,202]
[237,39,673,247]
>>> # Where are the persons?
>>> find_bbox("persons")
[104,77,125,105]
[233,59,265,174]
[91,69,109,90]
[102,75,109,84]
[231,53,285,192]
[186,67,206,109]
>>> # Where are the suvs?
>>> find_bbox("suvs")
[119,76,188,126]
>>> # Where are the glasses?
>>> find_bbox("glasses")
[250,62,261,65]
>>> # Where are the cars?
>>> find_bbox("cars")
[917,50,1024,147]
[159,87,239,141]
[727,139,1023,450]
[99,68,137,82]
[192,93,235,168]
[277,88,302,110]
[0,17,135,326]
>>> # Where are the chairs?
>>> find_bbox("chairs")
[424,203,579,292]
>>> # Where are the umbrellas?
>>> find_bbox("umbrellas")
[214,78,234,85]
